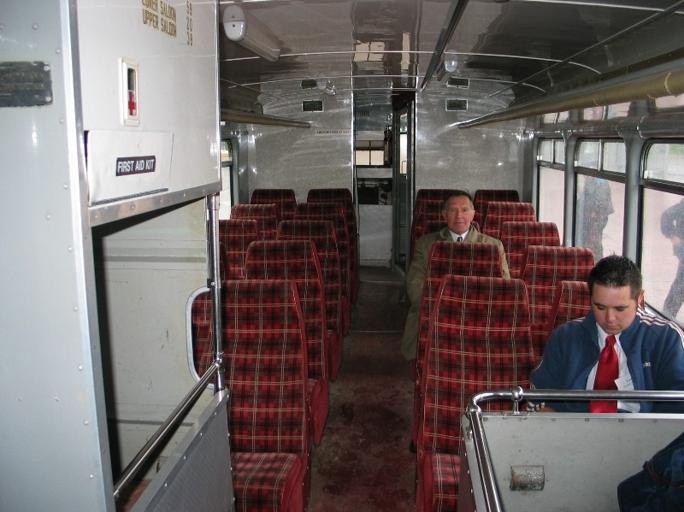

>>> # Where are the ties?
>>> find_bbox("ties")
[589,335,618,413]
[457,237,462,242]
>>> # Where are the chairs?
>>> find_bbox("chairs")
[413,188,644,509]
[191,188,356,512]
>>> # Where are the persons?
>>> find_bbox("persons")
[581,160,615,257]
[526,255,684,413]
[660,199,684,318]
[399,190,510,361]
[618,433,684,508]
